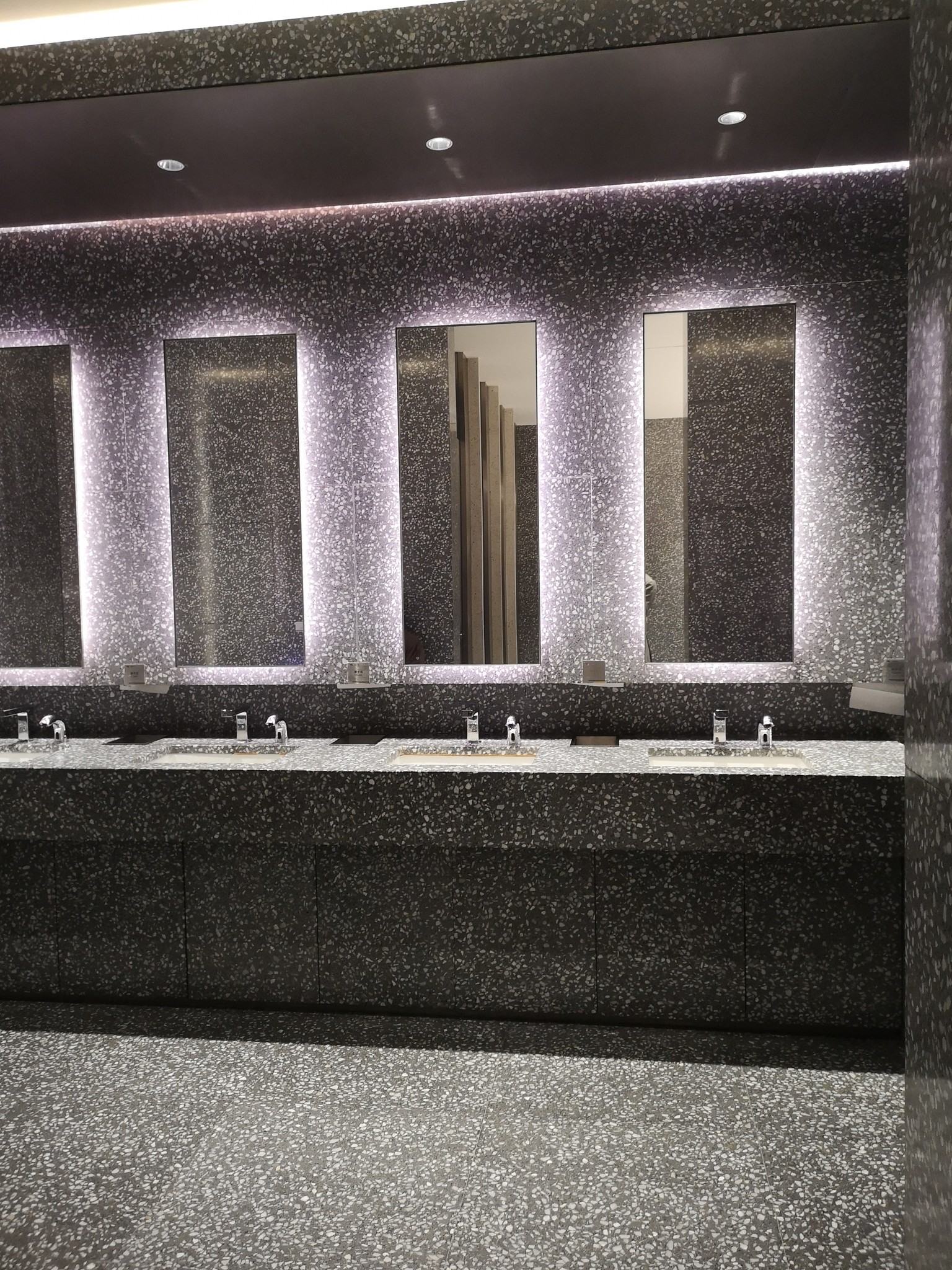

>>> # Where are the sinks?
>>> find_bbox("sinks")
[646,747,814,768]
[0,744,67,764]
[378,745,540,766]
[129,744,299,764]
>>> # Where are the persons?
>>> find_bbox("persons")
[645,573,657,662]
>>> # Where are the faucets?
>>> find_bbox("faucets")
[38,714,68,743]
[264,713,289,742]
[1,706,30,741]
[713,707,729,743]
[220,704,250,743]
[461,709,481,743]
[506,715,521,746]
[758,716,775,744]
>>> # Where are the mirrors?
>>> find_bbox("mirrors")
[157,330,313,670]
[0,341,85,669]
[641,296,801,667]
[393,320,543,673]
